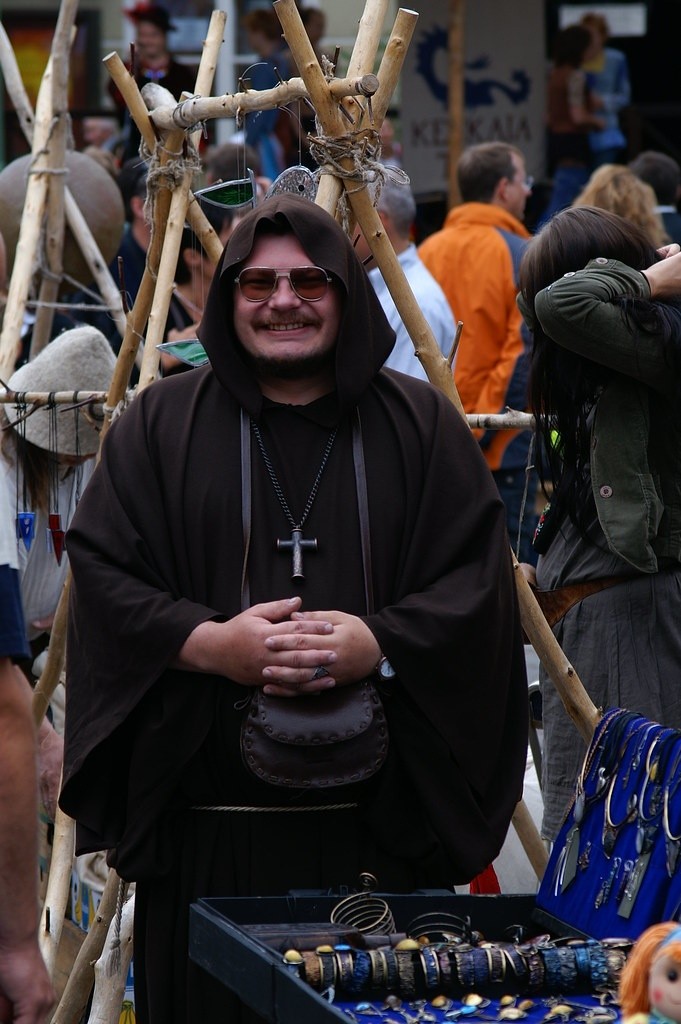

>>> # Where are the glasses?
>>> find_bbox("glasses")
[233,265,333,302]
[512,171,534,193]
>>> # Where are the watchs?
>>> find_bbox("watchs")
[375,656,396,680]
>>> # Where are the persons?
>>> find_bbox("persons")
[57,197,531,1024]
[345,166,458,385]
[574,165,665,249]
[628,150,681,247]
[619,923,681,1024]
[546,14,629,184]
[419,141,535,592]
[515,205,681,843]
[77,2,401,391]
[0,465,62,1024]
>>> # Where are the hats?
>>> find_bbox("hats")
[128,4,176,32]
[3,326,127,456]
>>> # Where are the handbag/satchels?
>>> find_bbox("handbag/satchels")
[240,682,388,788]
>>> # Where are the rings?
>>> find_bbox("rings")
[282,934,639,1024]
[311,665,330,680]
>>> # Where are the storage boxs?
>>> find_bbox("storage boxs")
[189,705,681,1024]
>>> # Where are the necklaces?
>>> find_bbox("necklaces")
[249,418,339,579]
[551,706,681,940]
